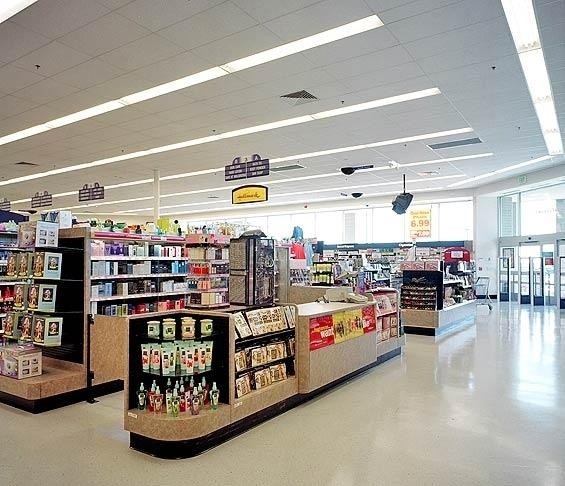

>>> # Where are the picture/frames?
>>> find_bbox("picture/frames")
[502,247,516,268]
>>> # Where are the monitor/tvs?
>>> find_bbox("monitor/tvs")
[392,193,413,214]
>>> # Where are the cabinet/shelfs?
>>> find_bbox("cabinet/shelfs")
[1,219,236,415]
[123,228,406,459]
[289,223,476,336]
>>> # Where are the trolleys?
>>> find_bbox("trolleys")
[472,276,493,310]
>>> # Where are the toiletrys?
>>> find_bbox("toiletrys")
[90,240,230,317]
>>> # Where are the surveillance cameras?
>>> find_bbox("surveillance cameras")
[341,168,355,175]
[352,193,362,198]
